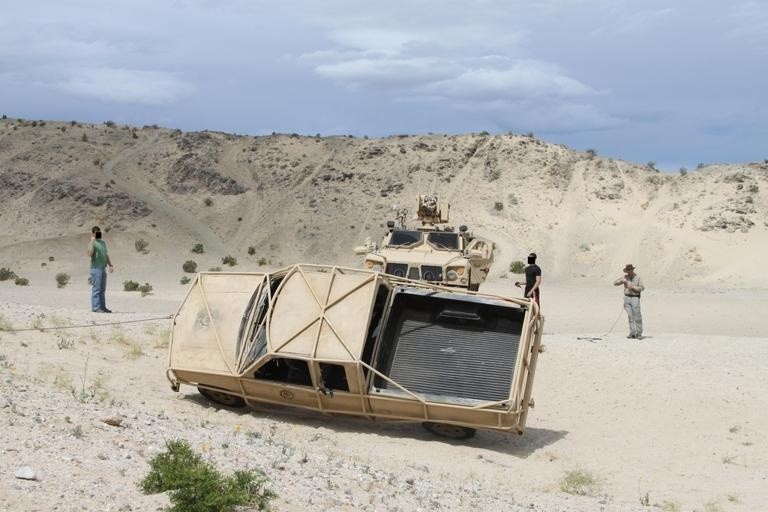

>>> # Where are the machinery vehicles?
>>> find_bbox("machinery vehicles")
[364,194,494,291]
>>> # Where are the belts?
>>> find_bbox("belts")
[625,294,639,297]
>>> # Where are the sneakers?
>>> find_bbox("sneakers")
[627,334,642,339]
[92,308,112,313]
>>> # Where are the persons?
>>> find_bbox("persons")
[613,265,644,340]
[514,253,541,307]
[87,227,114,313]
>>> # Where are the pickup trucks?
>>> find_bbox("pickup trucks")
[166,264,545,440]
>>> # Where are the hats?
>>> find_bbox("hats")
[623,264,636,273]
